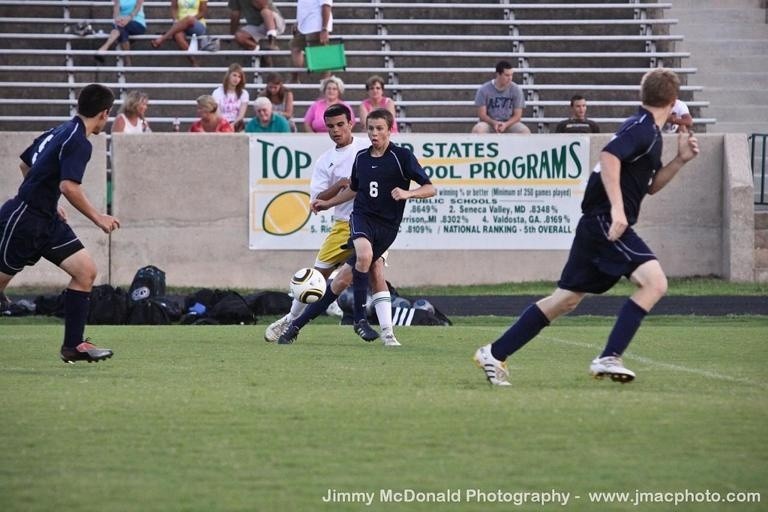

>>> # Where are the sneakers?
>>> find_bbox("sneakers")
[61,340,112,364]
[380,327,401,347]
[588,354,635,384]
[353,318,379,342]
[252,54,261,68]
[473,342,511,385]
[264,314,300,345]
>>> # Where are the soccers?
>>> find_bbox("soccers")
[290,267,328,304]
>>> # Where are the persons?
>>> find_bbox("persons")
[661,98,695,135]
[94,0,404,132]
[264,104,401,347]
[2,82,117,362]
[554,95,600,134]
[472,70,701,386]
[280,110,436,344]
[470,63,532,136]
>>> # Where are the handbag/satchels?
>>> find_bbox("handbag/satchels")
[368,303,454,326]
[35,266,291,326]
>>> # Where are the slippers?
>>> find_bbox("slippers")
[150,41,161,49]
